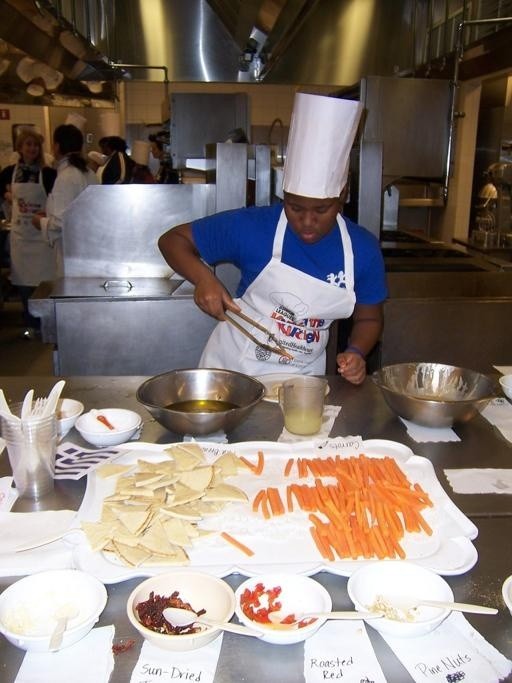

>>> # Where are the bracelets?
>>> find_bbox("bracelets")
[346,344,366,357]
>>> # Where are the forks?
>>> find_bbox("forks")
[31,397,47,419]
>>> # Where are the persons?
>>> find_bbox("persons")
[96,136,133,185]
[31,124,100,275]
[0,124,58,342]
[158,186,390,387]
[149,131,179,183]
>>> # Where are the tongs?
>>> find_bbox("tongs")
[223,305,287,357]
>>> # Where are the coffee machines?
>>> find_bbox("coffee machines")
[329,76,455,256]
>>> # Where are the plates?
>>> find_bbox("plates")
[2,506,74,557]
[253,372,330,405]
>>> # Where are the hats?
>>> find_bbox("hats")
[64,112,87,130]
[282,92,363,199]
[130,140,150,166]
[101,112,119,136]
[88,150,104,165]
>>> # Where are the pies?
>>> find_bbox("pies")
[81,444,248,569]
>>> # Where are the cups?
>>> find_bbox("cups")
[276,376,330,436]
[2,399,58,498]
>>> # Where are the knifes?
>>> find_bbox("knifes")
[20,389,35,418]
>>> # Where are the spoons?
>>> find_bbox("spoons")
[47,600,79,654]
[267,609,384,625]
[163,607,263,638]
[383,592,499,616]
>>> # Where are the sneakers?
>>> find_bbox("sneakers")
[15,326,36,344]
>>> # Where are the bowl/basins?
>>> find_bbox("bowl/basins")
[235,575,335,646]
[124,570,235,650]
[347,561,454,639]
[369,362,502,428]
[1,568,107,655]
[136,367,266,436]
[75,406,140,450]
[57,395,84,439]
[496,374,512,402]
[502,574,511,616]
